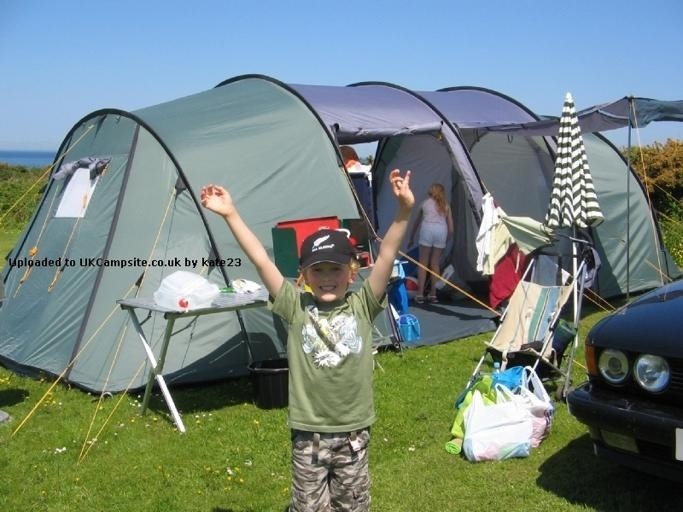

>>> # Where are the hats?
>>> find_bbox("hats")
[300,228,359,272]
[577,245,603,289]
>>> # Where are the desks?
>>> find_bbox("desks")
[116,287,269,433]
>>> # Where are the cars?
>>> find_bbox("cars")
[566,278,683,481]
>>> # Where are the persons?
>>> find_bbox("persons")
[196,169,412,512]
[408,183,455,303]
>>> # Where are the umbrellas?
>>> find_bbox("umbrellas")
[546,92,606,351]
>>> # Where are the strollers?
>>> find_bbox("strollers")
[457,226,594,404]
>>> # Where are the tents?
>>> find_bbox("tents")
[0,73,681,396]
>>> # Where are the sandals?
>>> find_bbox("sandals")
[414,293,425,305]
[424,293,440,303]
